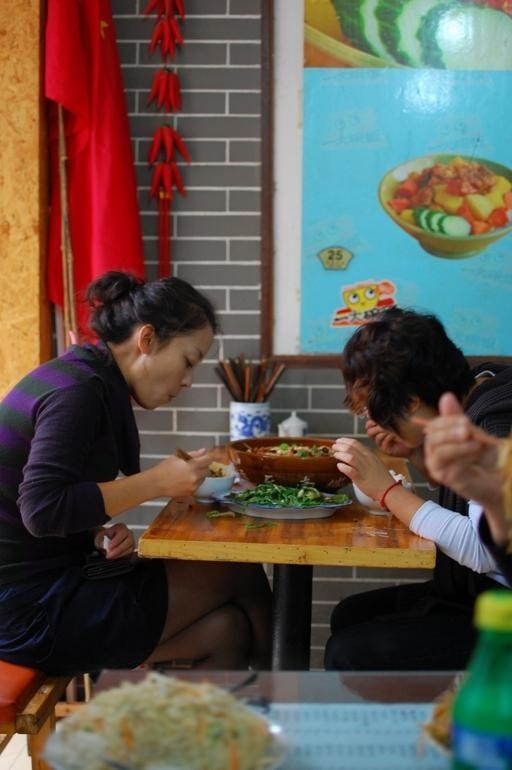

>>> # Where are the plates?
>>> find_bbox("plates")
[212,483,354,520]
[47,707,291,770]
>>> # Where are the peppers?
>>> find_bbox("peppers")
[144,0,190,200]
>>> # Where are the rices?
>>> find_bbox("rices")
[47,671,270,770]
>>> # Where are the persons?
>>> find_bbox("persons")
[1,272,275,684]
[323,307,512,671]
[422,390,512,587]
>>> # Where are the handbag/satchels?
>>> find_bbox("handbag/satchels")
[83,542,155,580]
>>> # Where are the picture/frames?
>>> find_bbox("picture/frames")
[260,0,512,368]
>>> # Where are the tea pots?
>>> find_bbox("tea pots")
[277,411,309,438]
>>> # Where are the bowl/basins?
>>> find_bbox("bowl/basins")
[224,438,361,494]
[349,475,411,516]
[379,154,510,260]
[196,464,234,504]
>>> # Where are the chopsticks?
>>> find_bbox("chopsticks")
[214,353,287,403]
[175,446,220,478]
[406,416,500,452]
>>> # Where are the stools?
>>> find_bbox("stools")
[0,661,95,770]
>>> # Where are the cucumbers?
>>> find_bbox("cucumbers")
[412,203,471,236]
[331,1,512,72]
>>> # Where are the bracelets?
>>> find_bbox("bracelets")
[380,479,406,513]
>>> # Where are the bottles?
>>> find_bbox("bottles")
[453,593,512,770]
